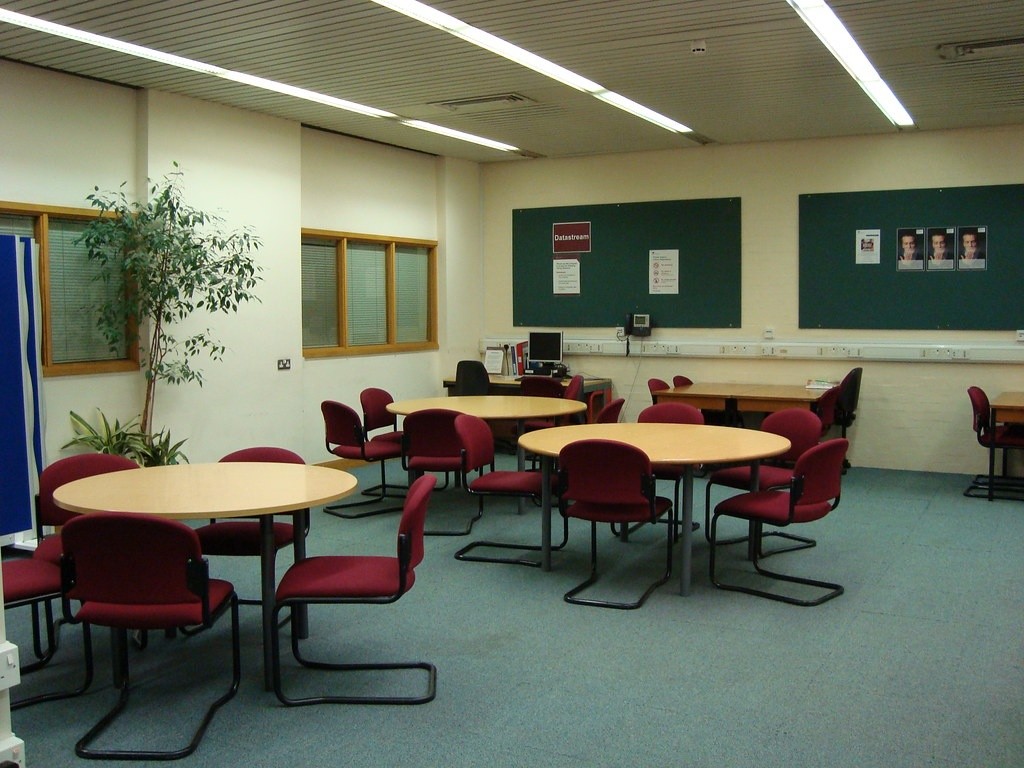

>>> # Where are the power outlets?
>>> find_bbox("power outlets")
[501,340,511,346]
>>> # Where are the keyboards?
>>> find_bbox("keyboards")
[516,376,562,381]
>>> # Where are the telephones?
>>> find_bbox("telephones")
[624,312,652,337]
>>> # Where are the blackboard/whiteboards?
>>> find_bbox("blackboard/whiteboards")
[798,183,1024,331]
[512,197,742,328]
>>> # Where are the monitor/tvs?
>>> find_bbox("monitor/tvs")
[528,330,563,361]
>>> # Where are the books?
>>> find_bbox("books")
[510,340,528,376]
[806,380,840,389]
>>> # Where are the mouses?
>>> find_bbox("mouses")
[563,376,572,378]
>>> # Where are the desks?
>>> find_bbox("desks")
[442,373,614,425]
[385,394,589,518]
[986,390,1024,501]
[650,380,839,430]
[516,421,793,600]
[50,458,360,700]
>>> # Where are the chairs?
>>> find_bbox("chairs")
[517,375,564,397]
[704,408,823,556]
[555,439,674,613]
[0,558,94,713]
[320,398,407,521]
[835,367,863,476]
[268,472,438,708]
[962,385,1024,502]
[519,374,585,462]
[186,446,311,637]
[454,360,492,398]
[454,414,569,570]
[359,387,450,502]
[30,451,150,628]
[594,397,626,424]
[810,386,841,441]
[56,511,242,763]
[401,409,484,539]
[672,374,695,388]
[706,436,850,609]
[609,400,706,543]
[648,378,669,407]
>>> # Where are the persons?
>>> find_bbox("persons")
[959,229,985,259]
[898,234,923,260]
[929,231,954,260]
[867,239,873,247]
[861,239,865,249]
